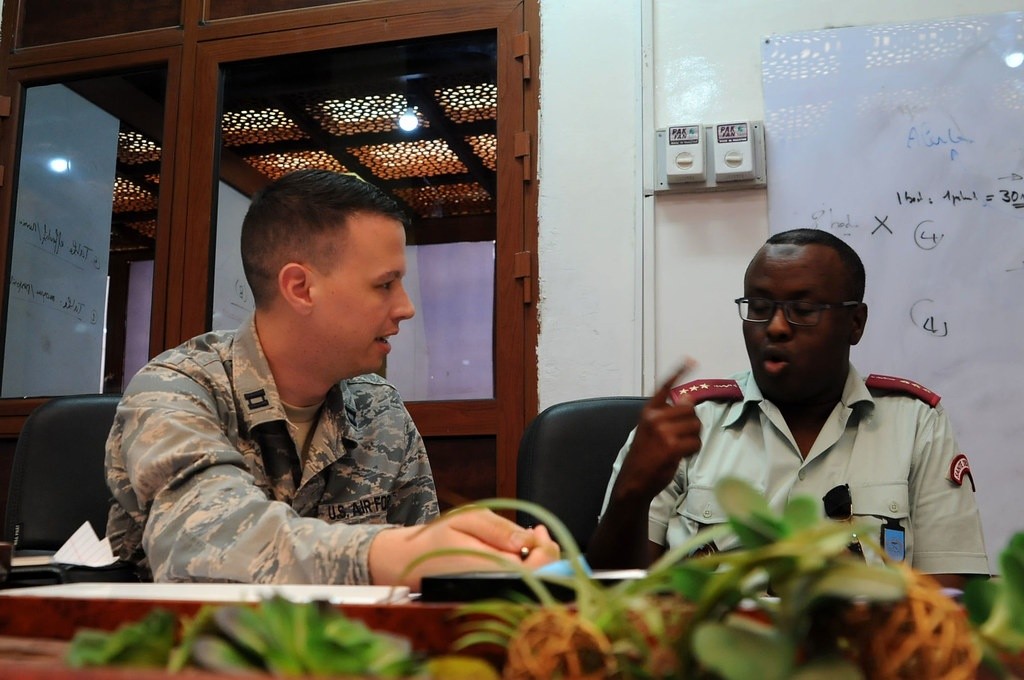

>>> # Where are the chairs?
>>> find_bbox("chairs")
[518,397,678,574]
[0,393,149,583]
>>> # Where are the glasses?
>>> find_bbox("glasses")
[734,296,859,327]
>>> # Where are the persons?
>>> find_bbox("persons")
[104,168,562,586]
[586,227,993,602]
